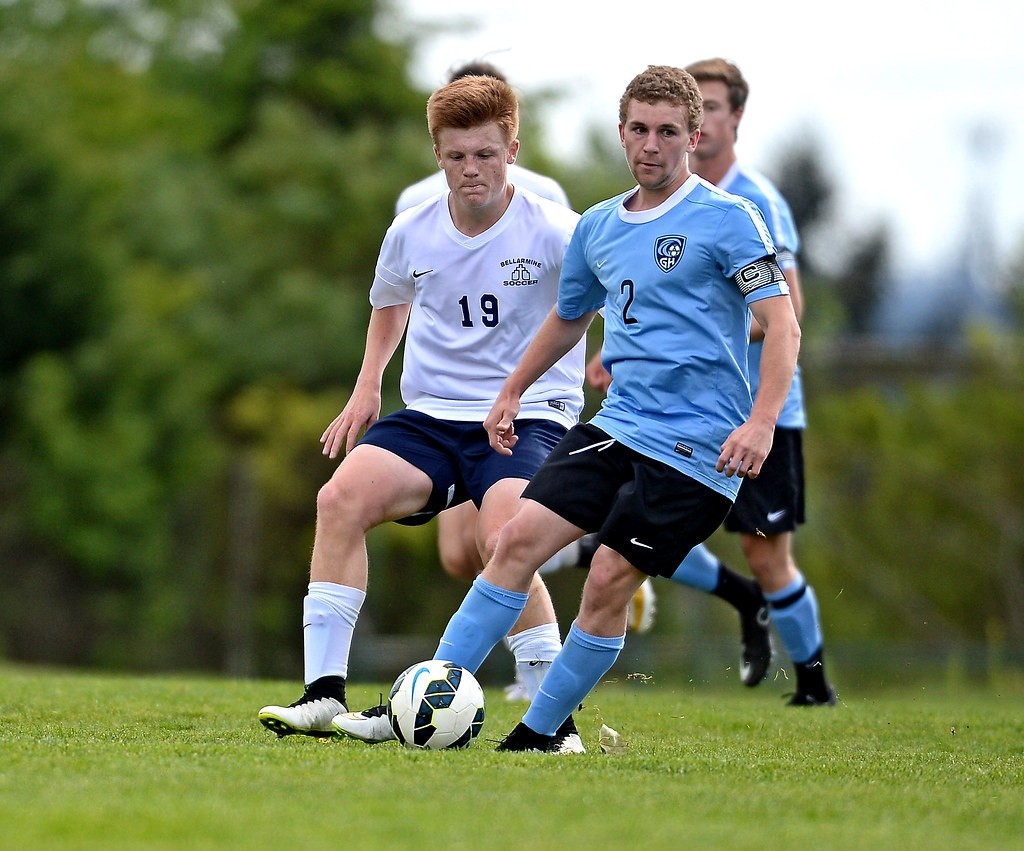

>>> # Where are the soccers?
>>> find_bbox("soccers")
[387,660,486,751]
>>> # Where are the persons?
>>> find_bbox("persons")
[259,57,836,756]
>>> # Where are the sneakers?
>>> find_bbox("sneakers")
[737,579,775,687]
[558,731,585,755]
[626,576,655,632]
[258,689,349,737]
[781,691,836,707]
[331,692,397,743]
[486,723,558,754]
